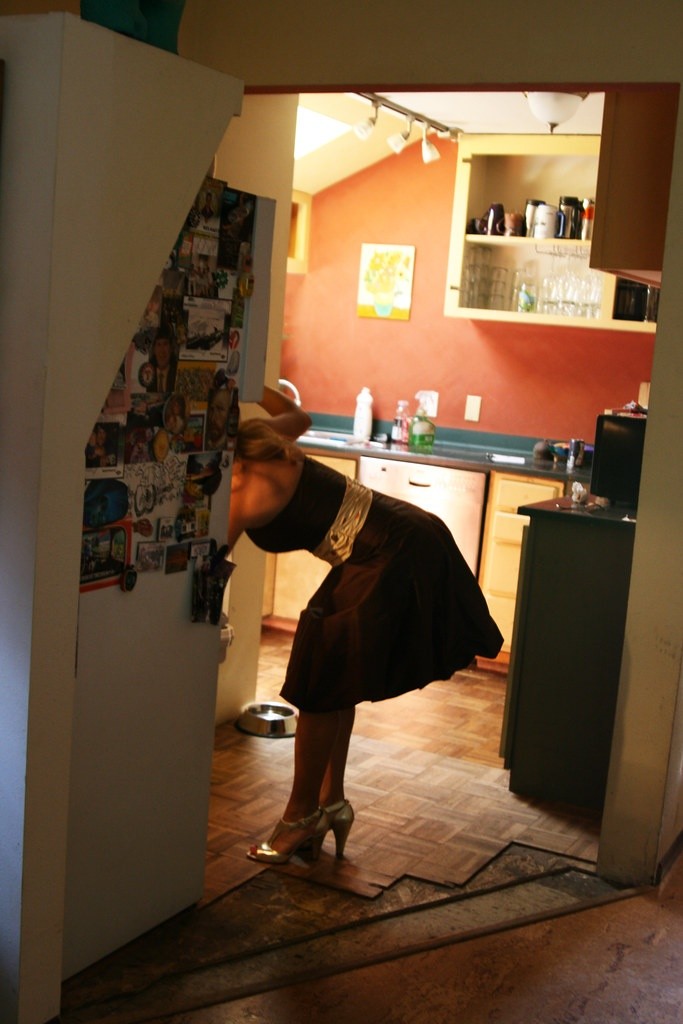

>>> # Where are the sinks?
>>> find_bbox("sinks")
[298,430,355,445]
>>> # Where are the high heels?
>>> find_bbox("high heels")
[298,799,354,859]
[245,806,329,864]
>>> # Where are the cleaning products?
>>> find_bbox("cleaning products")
[390,399,410,444]
[408,393,436,449]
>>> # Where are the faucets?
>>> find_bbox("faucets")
[279,377,302,408]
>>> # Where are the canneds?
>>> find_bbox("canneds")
[580,198,595,240]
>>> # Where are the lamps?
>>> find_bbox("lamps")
[350,103,380,140]
[419,122,441,164]
[523,91,587,134]
[387,112,414,153]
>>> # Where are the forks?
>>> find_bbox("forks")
[556,502,605,512]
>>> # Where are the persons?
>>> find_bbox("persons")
[201,192,214,219]
[228,386,505,864]
[205,389,234,452]
[141,362,154,387]
[85,425,112,467]
[147,332,177,394]
[167,399,183,432]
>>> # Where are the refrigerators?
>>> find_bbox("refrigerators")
[61,181,274,974]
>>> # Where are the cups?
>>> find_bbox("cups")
[462,246,602,320]
[468,194,594,240]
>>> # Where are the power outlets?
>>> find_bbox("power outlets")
[466,395,482,421]
[416,390,438,418]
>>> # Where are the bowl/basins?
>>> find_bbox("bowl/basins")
[235,702,299,738]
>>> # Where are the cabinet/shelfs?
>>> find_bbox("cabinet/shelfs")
[500,514,637,813]
[255,454,357,633]
[445,134,658,335]
[477,474,567,661]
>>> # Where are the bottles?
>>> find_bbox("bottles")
[353,386,373,440]
[390,400,411,452]
